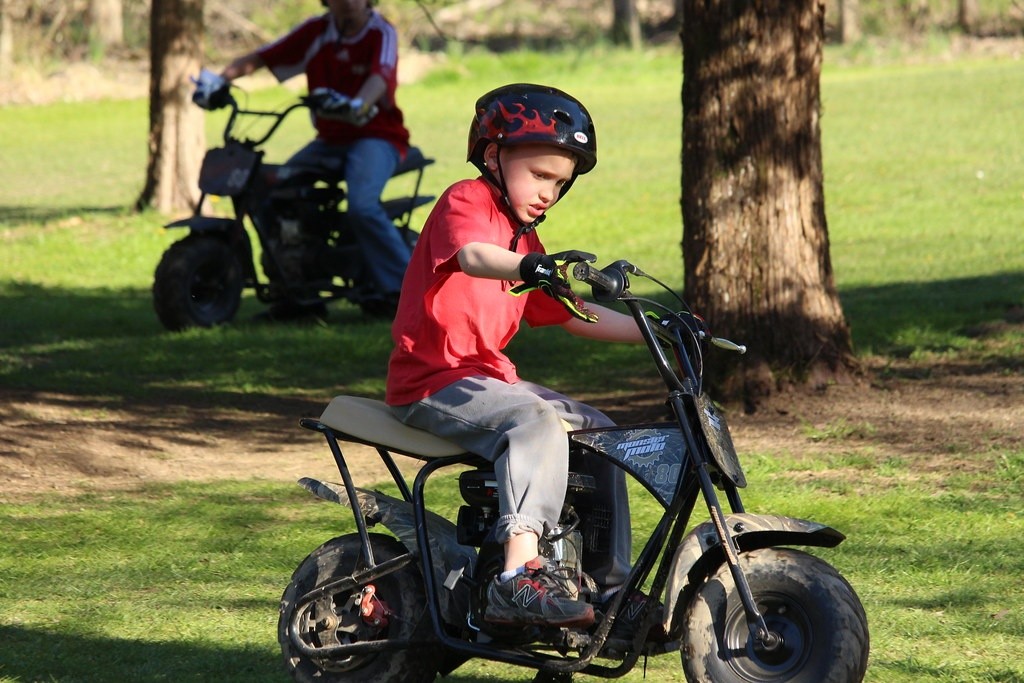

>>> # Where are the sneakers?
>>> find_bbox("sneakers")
[587,591,667,640]
[484,554,596,625]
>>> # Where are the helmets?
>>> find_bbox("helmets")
[319,0,381,10]
[466,83,598,173]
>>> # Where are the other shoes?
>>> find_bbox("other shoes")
[359,297,396,320]
[254,302,328,321]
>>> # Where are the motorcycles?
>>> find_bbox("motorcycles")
[153,72,441,337]
[274,256,872,682]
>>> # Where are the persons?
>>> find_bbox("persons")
[385,82,662,628]
[196,0,413,314]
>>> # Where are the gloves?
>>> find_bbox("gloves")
[518,250,609,325]
[192,65,226,109]
[313,86,363,123]
[647,311,710,353]
